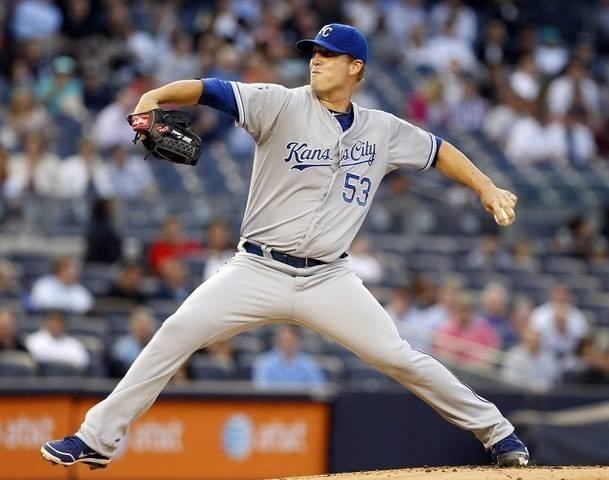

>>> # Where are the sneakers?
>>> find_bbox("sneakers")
[490,433,530,467]
[41,436,112,470]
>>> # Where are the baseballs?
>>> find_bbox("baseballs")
[494,207,515,226]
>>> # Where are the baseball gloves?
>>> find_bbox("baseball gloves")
[127,109,202,165]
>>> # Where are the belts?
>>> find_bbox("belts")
[245,242,325,268]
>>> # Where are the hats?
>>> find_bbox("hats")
[296,24,368,65]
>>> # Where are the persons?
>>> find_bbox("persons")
[1,0,609,396]
[40,23,532,474]
